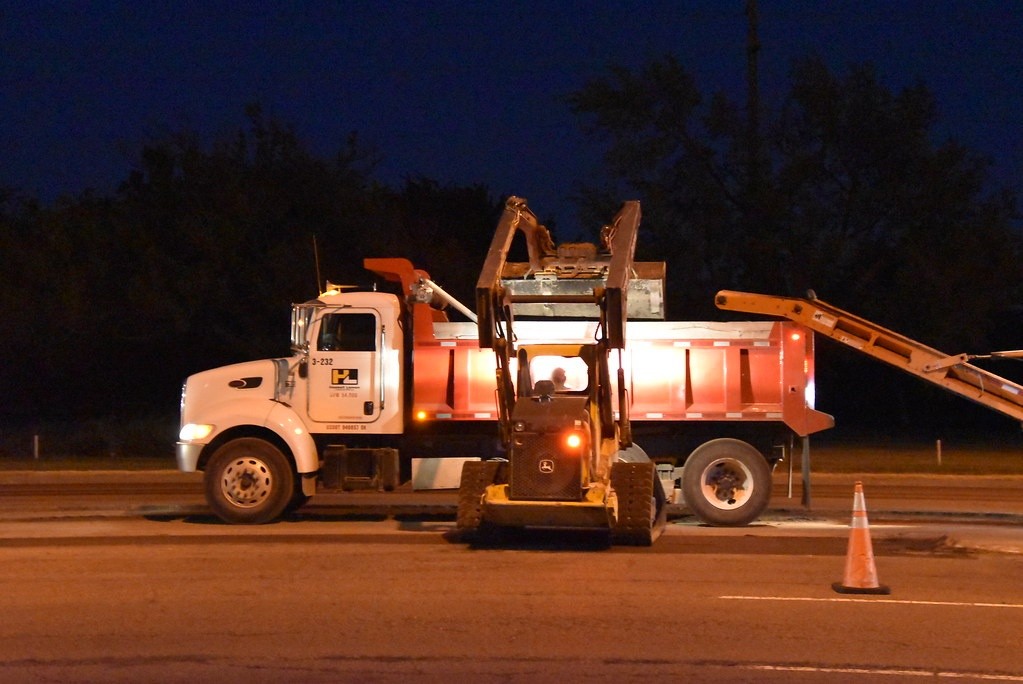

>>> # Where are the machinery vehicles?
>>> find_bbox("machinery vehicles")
[455,196,671,547]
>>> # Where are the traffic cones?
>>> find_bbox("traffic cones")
[832,480,891,595]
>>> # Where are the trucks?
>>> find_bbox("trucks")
[174,289,835,530]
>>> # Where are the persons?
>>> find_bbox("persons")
[551,367,571,391]
